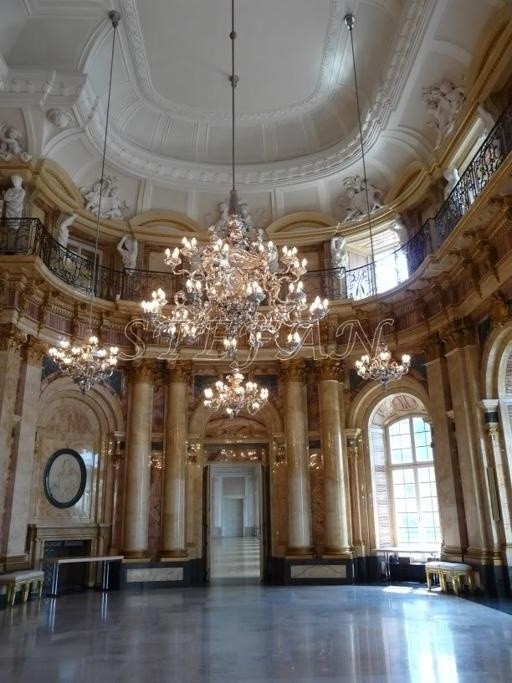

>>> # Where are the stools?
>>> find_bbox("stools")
[440,563,473,596]
[425,562,451,592]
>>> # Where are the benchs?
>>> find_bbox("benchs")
[0,570,44,606]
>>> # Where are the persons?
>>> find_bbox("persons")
[116,235,139,276]
[426,87,451,152]
[215,201,229,229]
[50,458,80,503]
[241,202,256,229]
[346,187,364,218]
[5,174,27,228]
[441,81,466,119]
[85,180,105,213]
[2,124,23,161]
[359,180,385,211]
[60,211,79,257]
[107,179,122,219]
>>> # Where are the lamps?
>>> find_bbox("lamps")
[48,1,410,421]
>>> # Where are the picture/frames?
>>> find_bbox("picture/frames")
[43,448,87,509]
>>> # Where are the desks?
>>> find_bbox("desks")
[48,555,125,597]
